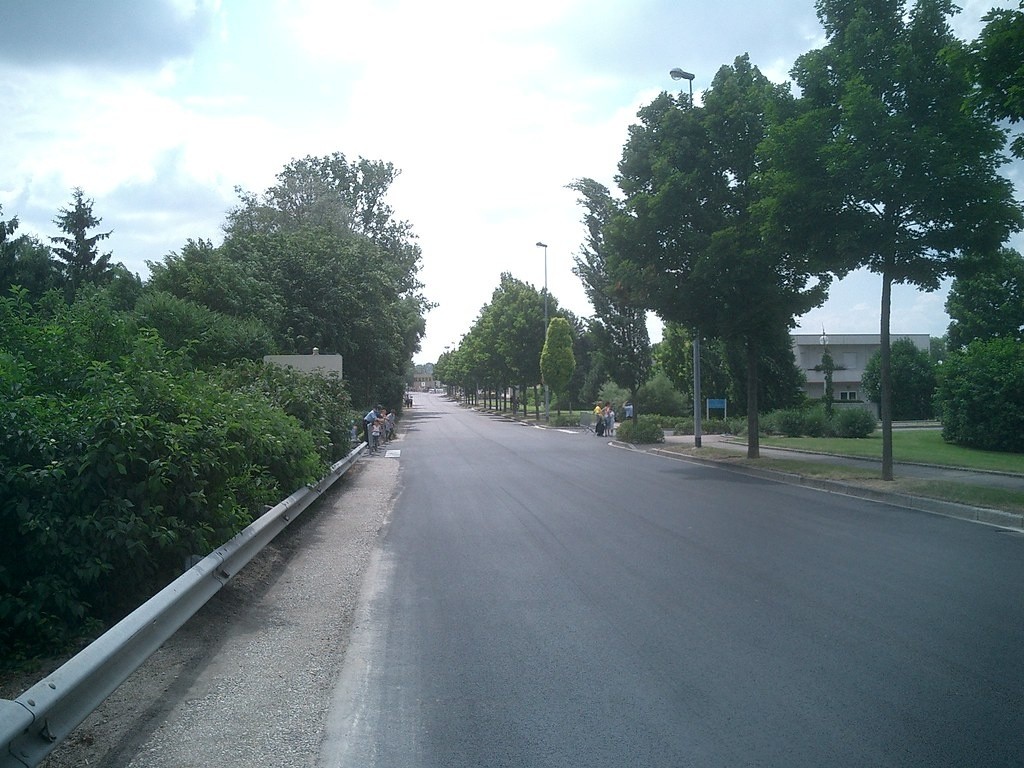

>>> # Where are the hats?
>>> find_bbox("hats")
[373,406,378,409]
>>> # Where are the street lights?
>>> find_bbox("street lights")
[668,67,703,447]
[535,242,551,423]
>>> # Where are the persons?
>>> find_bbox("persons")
[362,404,396,455]
[594,401,616,438]
[405,393,414,408]
[622,400,633,420]
